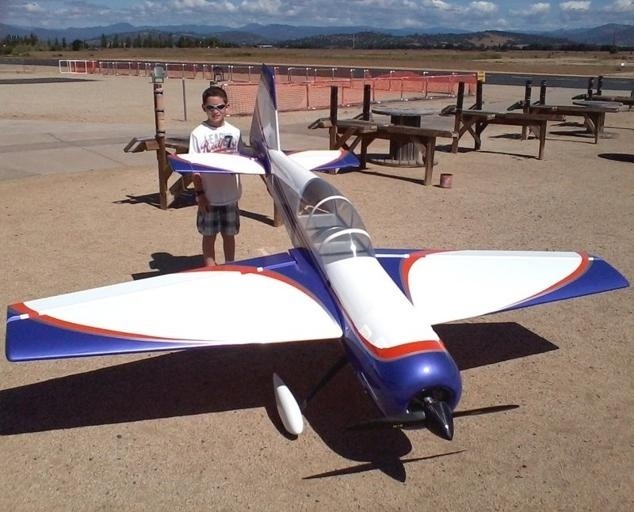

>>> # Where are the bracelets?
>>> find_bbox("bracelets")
[193,191,204,197]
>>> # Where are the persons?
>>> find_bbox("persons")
[185,86,243,266]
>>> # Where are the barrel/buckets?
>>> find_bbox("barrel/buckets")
[440,173,454,188]
